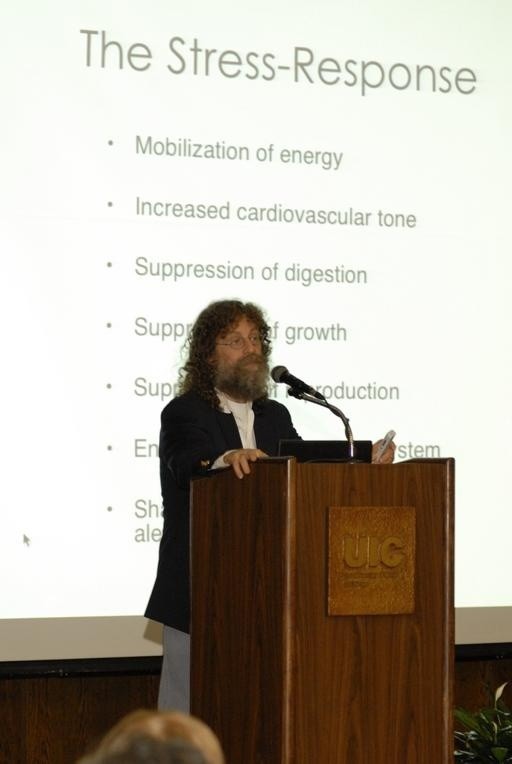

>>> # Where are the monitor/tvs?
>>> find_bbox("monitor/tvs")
[278,439,372,463]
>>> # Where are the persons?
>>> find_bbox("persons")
[78,706,227,764]
[142,298,395,722]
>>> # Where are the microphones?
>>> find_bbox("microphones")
[271,366,325,401]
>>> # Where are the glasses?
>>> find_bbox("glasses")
[215,334,263,350]
